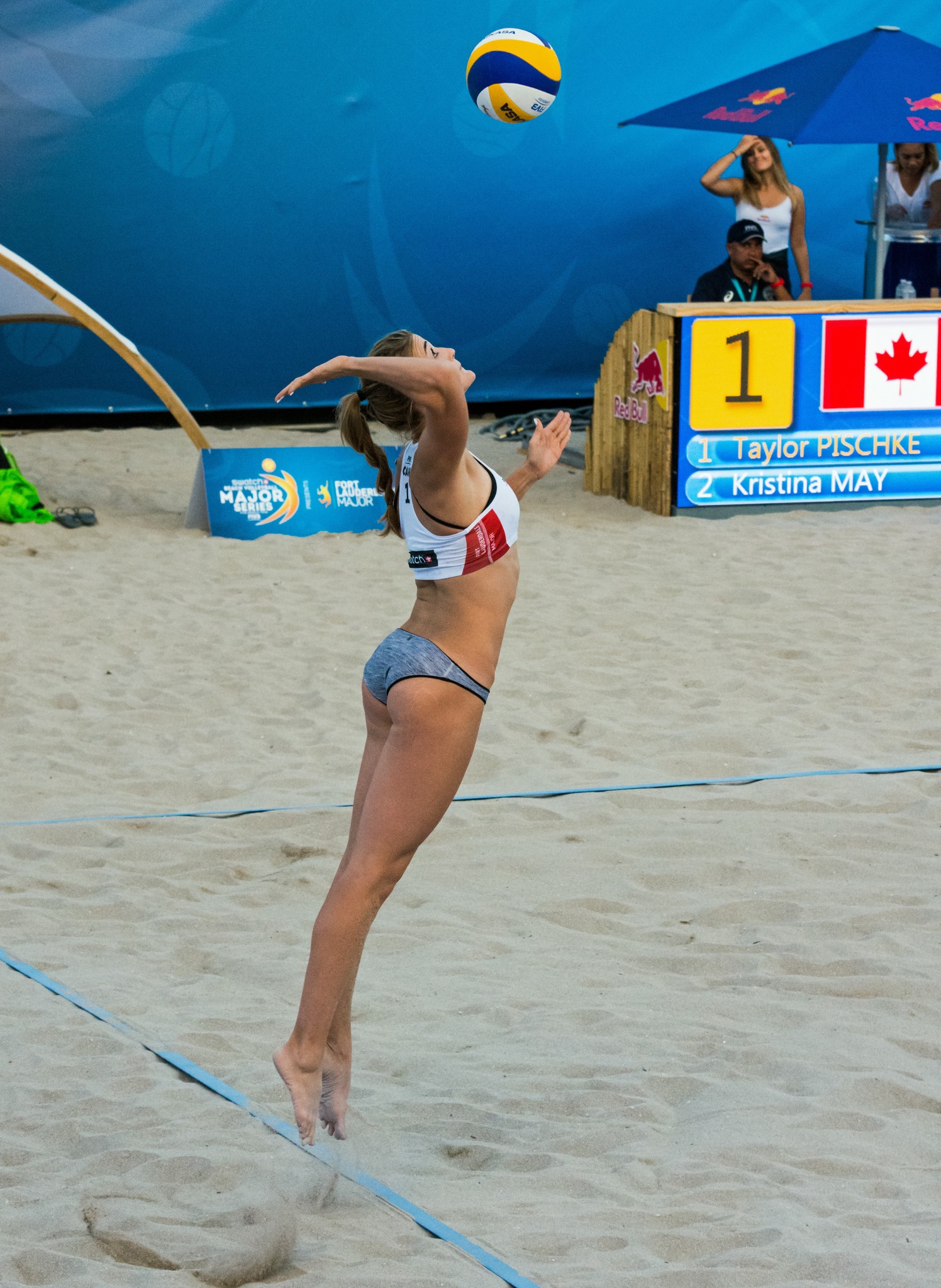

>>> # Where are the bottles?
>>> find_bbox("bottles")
[901,281,916,298]
[895,280,906,298]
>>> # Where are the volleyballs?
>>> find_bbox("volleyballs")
[465,28,560,123]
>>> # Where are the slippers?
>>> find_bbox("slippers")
[54,506,98,529]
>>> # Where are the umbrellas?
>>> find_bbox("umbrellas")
[618,27,941,301]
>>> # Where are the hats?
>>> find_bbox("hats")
[727,220,768,246]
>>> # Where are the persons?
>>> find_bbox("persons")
[872,143,941,299]
[272,329,573,1147]
[692,219,794,301]
[701,135,813,301]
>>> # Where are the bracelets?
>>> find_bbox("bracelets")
[731,151,739,159]
[771,277,785,289]
[800,282,813,288]
